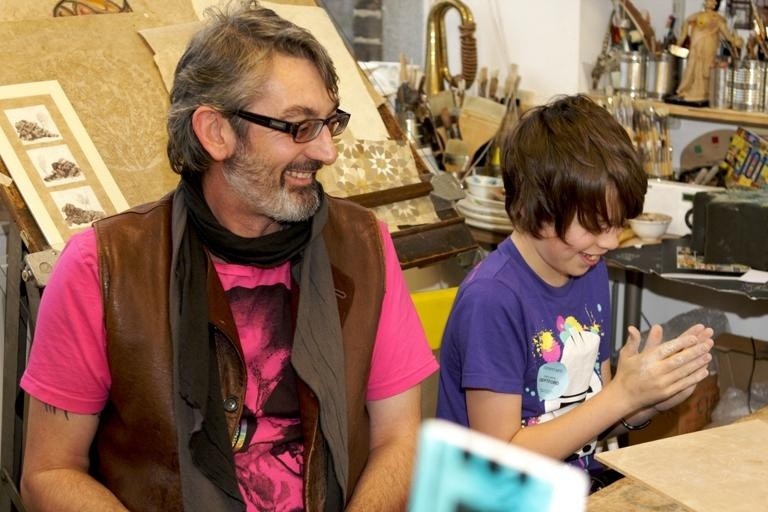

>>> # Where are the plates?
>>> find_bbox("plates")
[455,199,515,232]
[467,190,480,199]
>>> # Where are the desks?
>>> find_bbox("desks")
[468,218,768,348]
[581,403,767,512]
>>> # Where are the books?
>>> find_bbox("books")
[656,236,752,282]
[717,125,768,195]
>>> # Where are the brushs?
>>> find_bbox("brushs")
[442,138,471,175]
[426,89,460,116]
[458,93,507,164]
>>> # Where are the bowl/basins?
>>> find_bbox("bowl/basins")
[464,175,505,199]
[628,214,673,243]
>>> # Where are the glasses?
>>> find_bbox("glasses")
[236,108,352,144]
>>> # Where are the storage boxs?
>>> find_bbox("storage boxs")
[623,369,722,446]
[642,179,727,237]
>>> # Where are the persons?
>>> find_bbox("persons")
[665,0,740,105]
[430,91,717,501]
[17,0,443,511]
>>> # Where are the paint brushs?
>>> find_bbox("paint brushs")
[422,108,462,172]
[399,53,427,96]
[604,88,672,178]
[478,63,521,131]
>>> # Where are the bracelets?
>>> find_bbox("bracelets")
[617,418,653,433]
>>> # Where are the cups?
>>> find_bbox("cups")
[618,51,679,100]
